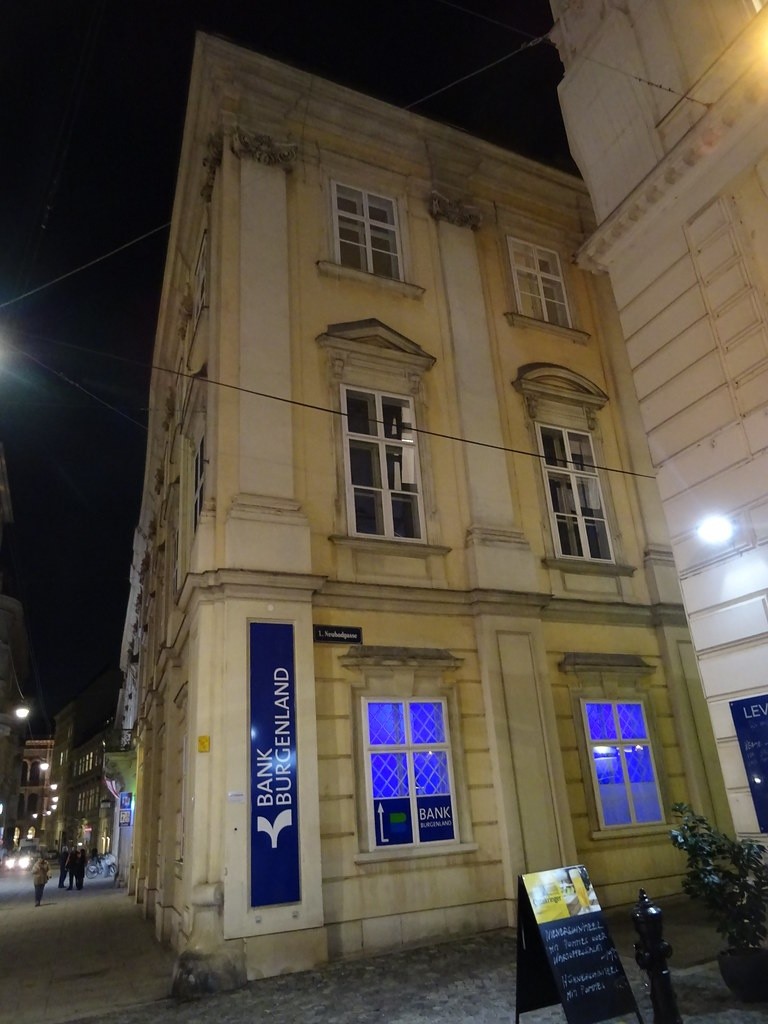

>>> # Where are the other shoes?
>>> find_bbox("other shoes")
[60,886,65,888]
[67,888,71,890]
[35,902,40,906]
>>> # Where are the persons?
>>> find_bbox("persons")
[59,845,101,890]
[32,854,52,908]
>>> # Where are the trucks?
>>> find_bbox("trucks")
[19,838,41,860]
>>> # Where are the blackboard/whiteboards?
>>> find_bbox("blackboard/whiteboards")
[537,911,637,1024]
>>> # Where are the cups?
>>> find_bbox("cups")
[569,870,589,907]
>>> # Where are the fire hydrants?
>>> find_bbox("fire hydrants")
[630,889,685,1024]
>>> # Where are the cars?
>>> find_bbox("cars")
[47,849,58,858]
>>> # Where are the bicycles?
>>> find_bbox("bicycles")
[86,855,115,878]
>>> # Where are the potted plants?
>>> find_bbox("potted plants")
[668,801,768,1004]
